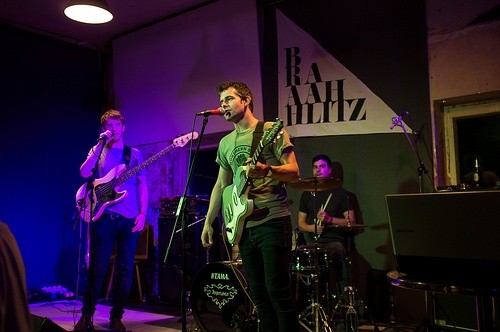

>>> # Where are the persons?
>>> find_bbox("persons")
[200,81,300,332]
[297,154,356,256]
[73,109,149,332]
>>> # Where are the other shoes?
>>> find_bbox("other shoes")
[74,314,92,332]
[109,318,126,332]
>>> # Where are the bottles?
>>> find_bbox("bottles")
[472,159,480,191]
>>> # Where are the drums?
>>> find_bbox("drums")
[189,260,253,332]
[288,245,335,275]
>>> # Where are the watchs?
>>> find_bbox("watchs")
[265,166,273,177]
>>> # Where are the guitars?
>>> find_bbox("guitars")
[221,117,285,246]
[75,129,201,224]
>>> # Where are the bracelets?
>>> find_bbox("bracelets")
[140,212,146,217]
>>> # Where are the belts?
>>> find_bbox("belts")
[102,213,122,219]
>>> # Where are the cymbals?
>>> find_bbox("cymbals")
[325,221,375,230]
[195,198,210,205]
[289,177,343,193]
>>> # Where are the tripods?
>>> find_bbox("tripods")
[298,179,334,332]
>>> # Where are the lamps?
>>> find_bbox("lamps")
[64,0,113,24]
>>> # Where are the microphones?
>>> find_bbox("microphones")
[99,131,114,140]
[389,111,409,129]
[197,107,225,116]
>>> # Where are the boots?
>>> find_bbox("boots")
[259,310,279,332]
[274,302,297,332]
